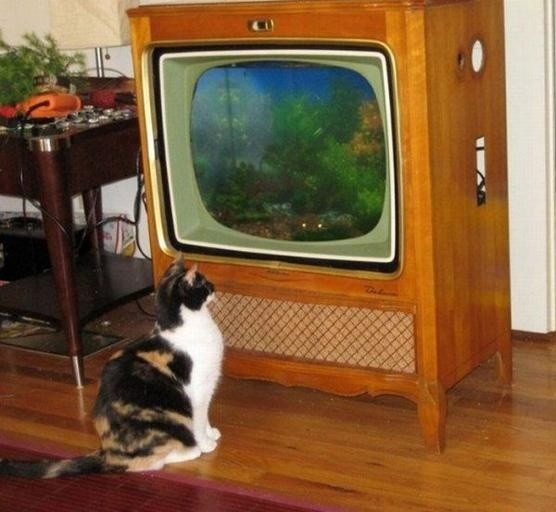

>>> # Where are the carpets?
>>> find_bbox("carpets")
[0,430,345,512]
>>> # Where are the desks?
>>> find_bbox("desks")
[0,77,151,392]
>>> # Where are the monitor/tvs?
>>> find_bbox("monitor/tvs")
[157,48,397,266]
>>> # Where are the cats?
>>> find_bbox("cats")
[0,249,227,478]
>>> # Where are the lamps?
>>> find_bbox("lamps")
[49,0,138,78]
